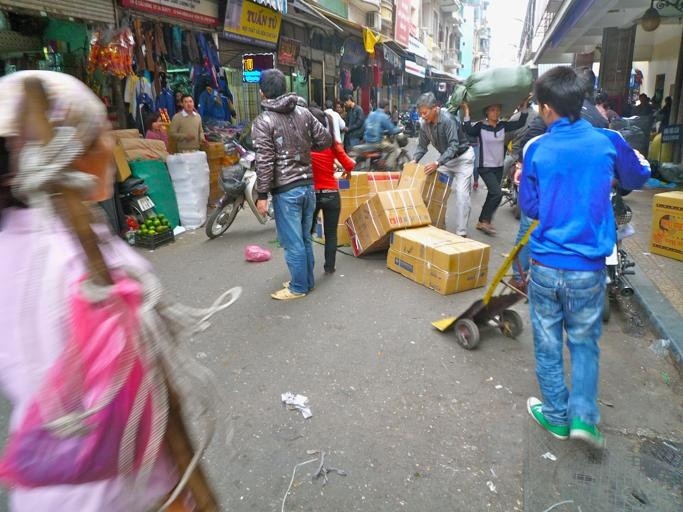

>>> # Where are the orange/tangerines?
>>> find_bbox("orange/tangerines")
[136,214,171,235]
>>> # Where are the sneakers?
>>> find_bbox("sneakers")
[477,222,496,235]
[527,397,605,447]
[271,281,314,299]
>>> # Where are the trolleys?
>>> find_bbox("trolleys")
[434,215,537,352]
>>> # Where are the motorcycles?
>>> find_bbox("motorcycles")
[205,140,277,240]
[214,127,256,164]
[497,155,521,217]
[394,105,422,138]
[602,182,639,326]
[347,130,410,175]
[115,176,156,235]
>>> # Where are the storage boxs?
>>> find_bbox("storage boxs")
[311,161,490,296]
[201,141,224,202]
[648,188,682,261]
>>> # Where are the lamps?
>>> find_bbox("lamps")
[641,1,683,34]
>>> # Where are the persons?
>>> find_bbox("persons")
[0,70,203,512]
[517,66,651,450]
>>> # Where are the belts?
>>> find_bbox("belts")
[316,190,337,194]
